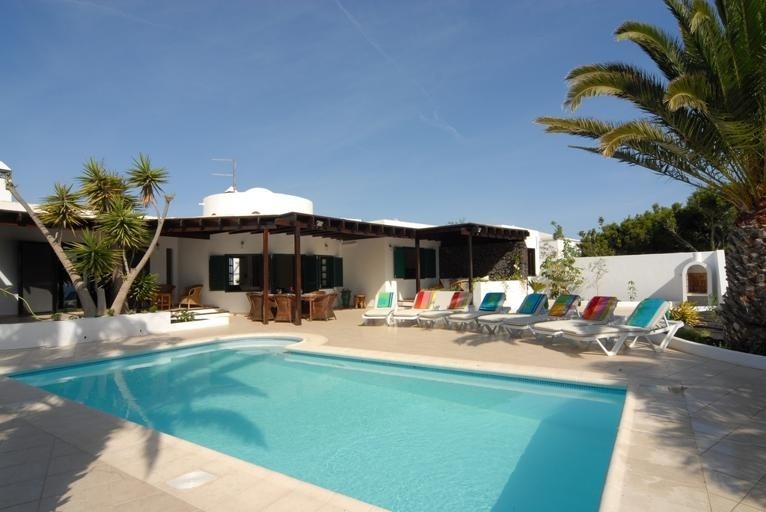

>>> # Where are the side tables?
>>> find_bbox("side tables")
[355,294,367,308]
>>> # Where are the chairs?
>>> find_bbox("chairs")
[476,293,549,335]
[156,284,204,309]
[562,298,684,357]
[395,292,434,327]
[501,294,581,338]
[363,290,398,327]
[416,291,471,328]
[246,291,339,325]
[449,293,511,335]
[535,295,625,344]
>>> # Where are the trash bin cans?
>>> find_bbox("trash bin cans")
[340,289,351,308]
